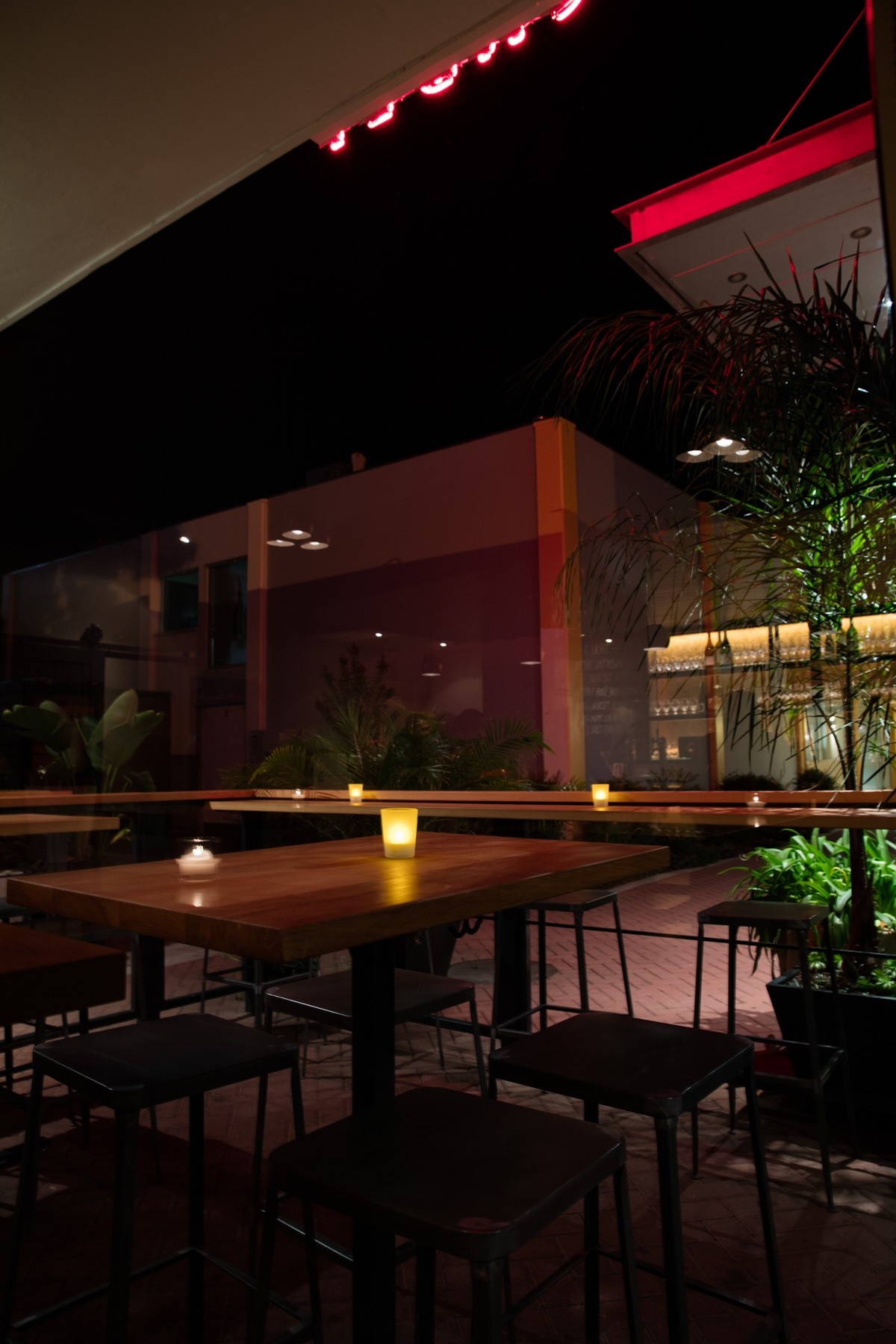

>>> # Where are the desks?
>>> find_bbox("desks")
[5,830,670,1344]
[1,813,120,838]
[0,922,127,1027]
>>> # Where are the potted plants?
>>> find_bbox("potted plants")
[705,828,896,979]
[218,649,585,977]
[0,692,165,944]
[549,231,896,1162]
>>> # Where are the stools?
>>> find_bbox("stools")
[0,884,843,1344]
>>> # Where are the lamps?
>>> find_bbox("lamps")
[673,304,764,467]
